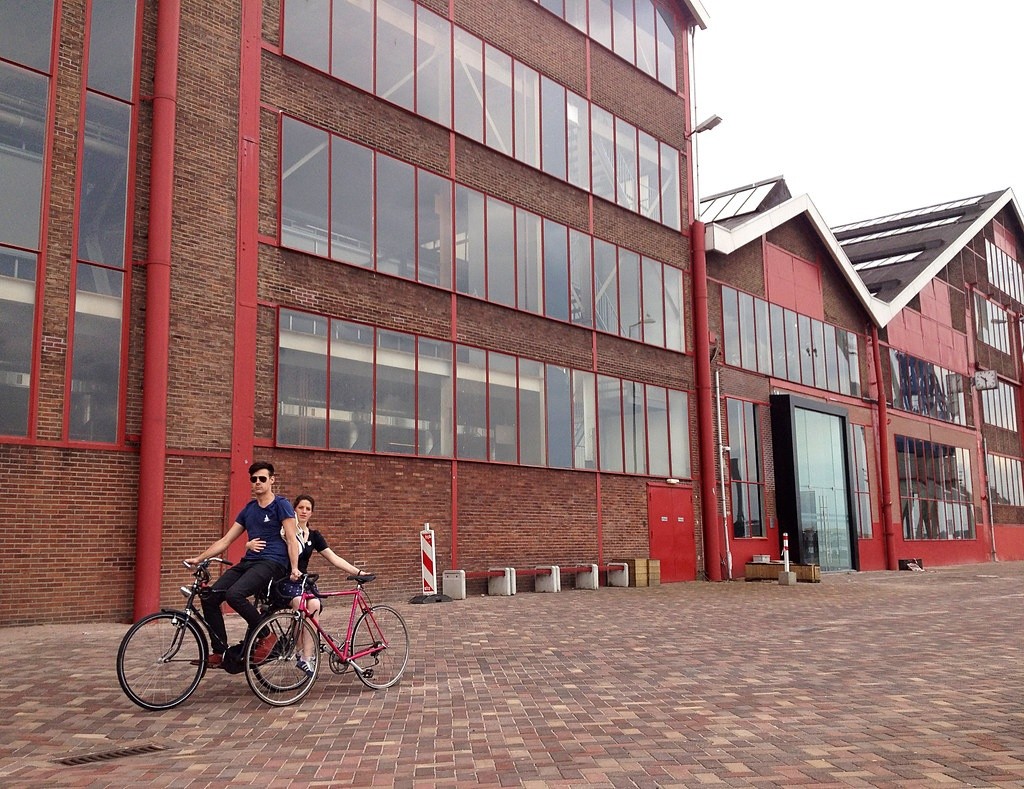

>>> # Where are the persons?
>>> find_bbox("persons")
[184,461,299,668]
[278,494,371,679]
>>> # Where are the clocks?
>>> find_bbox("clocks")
[946,373,963,394]
[973,369,1000,390]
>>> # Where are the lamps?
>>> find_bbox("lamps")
[688,115,721,137]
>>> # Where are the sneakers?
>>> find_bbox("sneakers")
[190,653,222,669]
[252,633,278,664]
[295,650,315,675]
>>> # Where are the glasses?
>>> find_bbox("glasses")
[249,475,271,483]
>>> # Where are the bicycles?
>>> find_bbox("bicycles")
[243,574,410,707]
[116,557,322,712]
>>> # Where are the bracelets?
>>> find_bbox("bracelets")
[357,570,361,576]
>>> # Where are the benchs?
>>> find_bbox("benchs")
[745,562,821,583]
